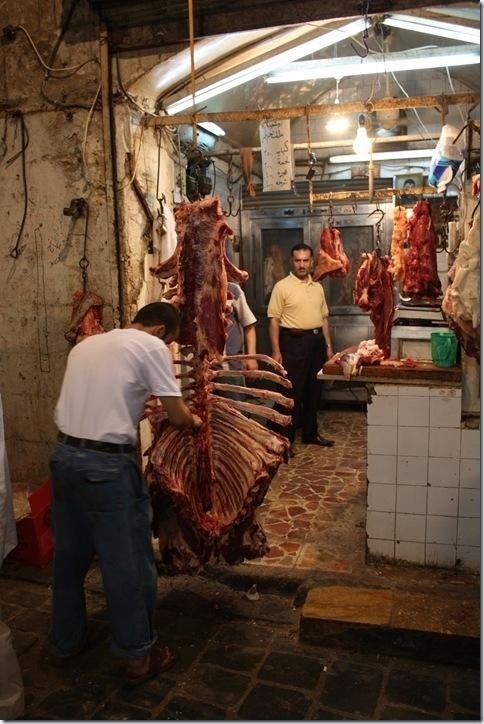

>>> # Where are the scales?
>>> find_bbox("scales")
[392,293,445,326]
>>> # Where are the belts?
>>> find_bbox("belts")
[281,327,321,336]
[56,431,135,455]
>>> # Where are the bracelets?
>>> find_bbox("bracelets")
[324,343,333,348]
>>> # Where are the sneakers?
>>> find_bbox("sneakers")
[122,637,177,685]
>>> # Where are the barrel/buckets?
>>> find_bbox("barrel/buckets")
[430,332,458,367]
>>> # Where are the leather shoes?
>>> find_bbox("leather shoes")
[284,432,335,448]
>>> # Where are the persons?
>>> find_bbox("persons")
[223,278,260,416]
[265,242,336,446]
[41,300,204,689]
